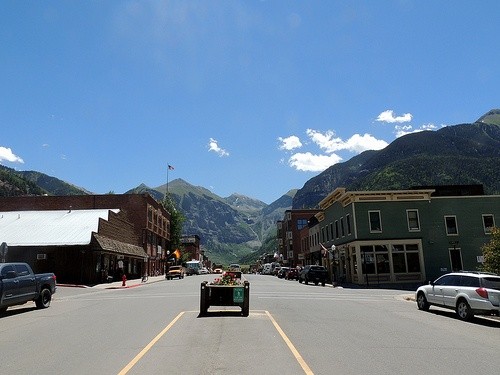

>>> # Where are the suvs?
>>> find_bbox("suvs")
[415,271,500,321]
[166,265,184,280]
[299,265,328,286]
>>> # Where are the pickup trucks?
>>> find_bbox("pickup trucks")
[0,263,57,312]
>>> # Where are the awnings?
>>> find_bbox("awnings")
[93,235,148,259]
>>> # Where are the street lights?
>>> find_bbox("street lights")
[331,245,337,286]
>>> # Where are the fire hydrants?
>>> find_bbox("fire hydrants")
[122,274,127,285]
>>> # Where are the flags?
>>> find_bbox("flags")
[173,248,180,259]
[168,164,174,170]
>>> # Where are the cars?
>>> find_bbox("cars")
[186,266,223,275]
[262,262,289,278]
[285,268,299,281]
